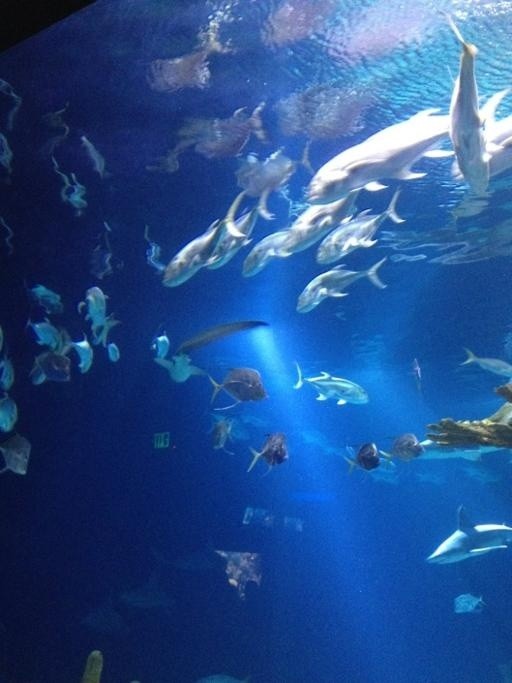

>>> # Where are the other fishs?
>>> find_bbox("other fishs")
[0,11,512,478]
[453,593,485,613]
